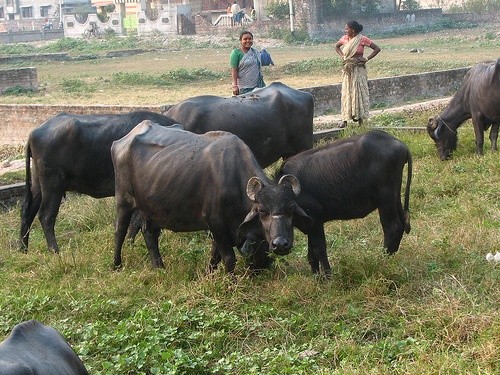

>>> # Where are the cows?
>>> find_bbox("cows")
[426,57,500,162]
[20,111,182,254]
[164,81,314,169]
[238,129,413,278]
[0,319,90,375]
[111,119,273,278]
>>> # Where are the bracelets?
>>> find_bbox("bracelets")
[232,85,238,88]
[365,57,368,61]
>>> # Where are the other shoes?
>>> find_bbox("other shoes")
[339,120,347,128]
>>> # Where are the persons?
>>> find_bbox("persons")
[335,21,381,128]
[226,0,257,20]
[230,31,266,95]
[259,47,274,66]
